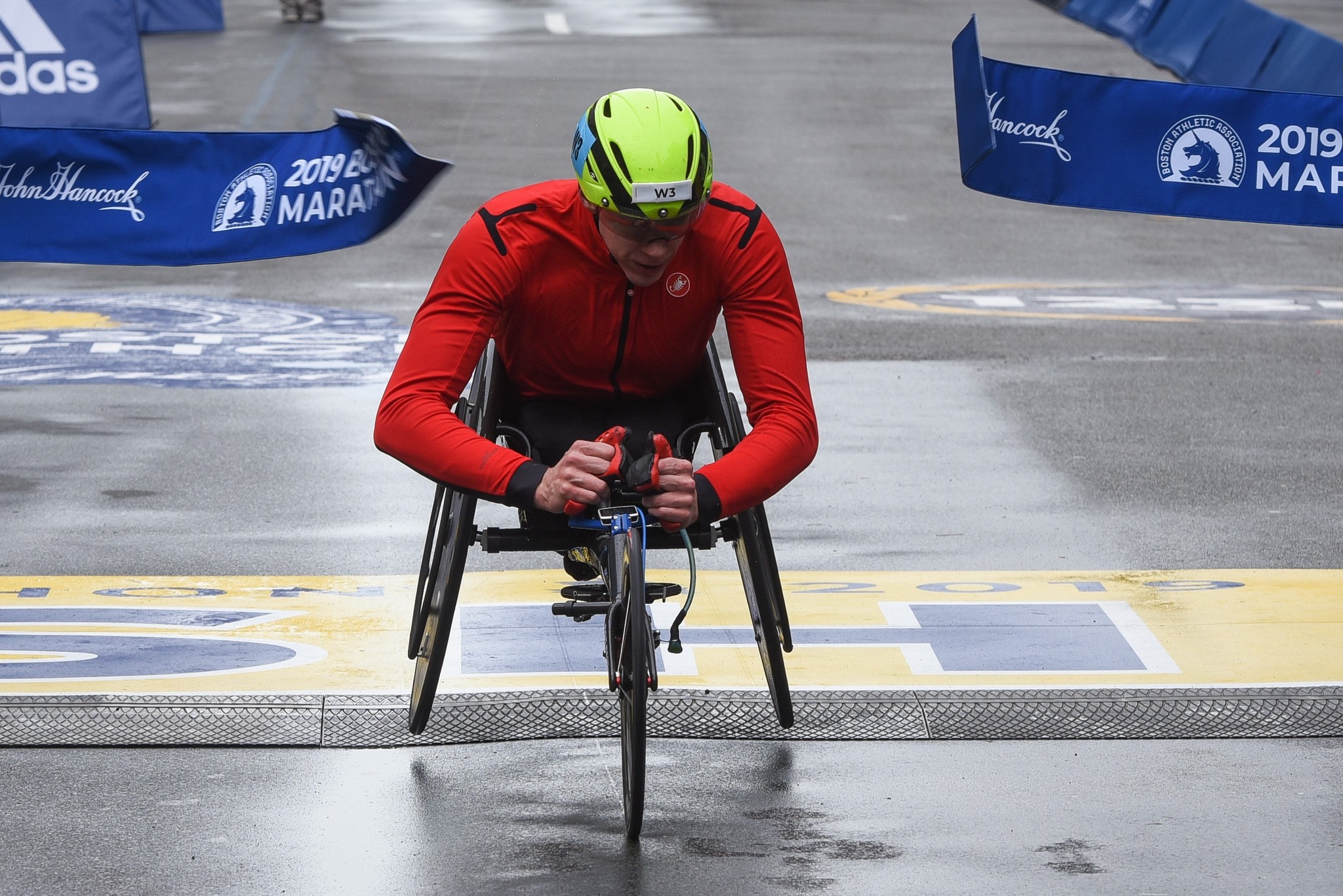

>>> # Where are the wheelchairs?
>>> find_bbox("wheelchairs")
[408,333,794,839]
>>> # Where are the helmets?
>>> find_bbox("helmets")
[570,88,713,242]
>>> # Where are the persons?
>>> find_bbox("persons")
[373,88,819,531]
